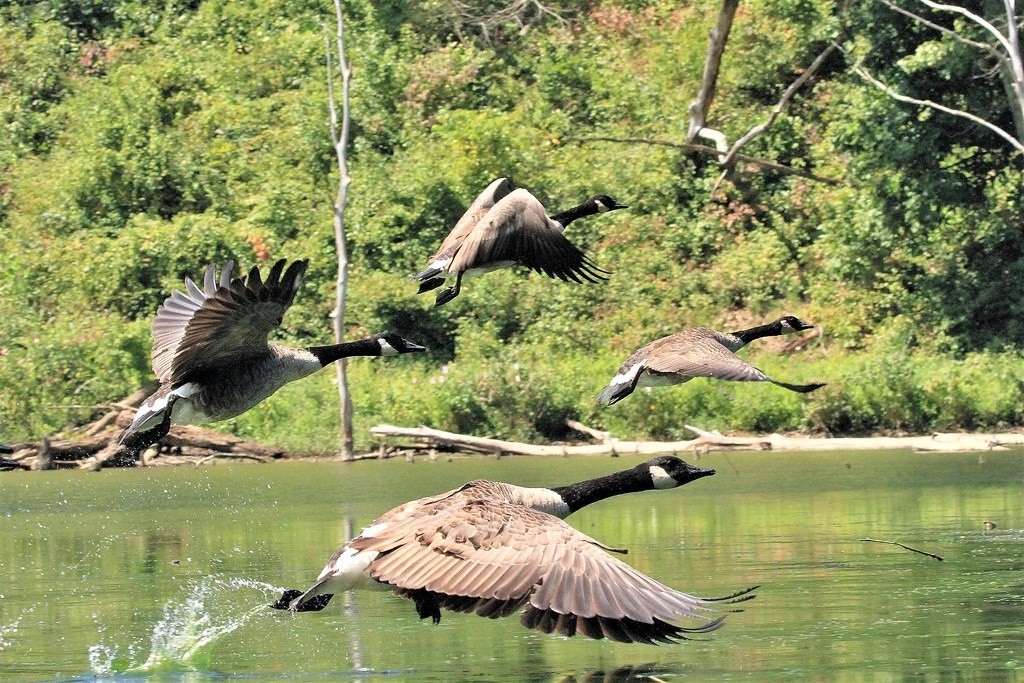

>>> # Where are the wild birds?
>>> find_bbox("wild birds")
[597,316,827,407]
[115,258,428,447]
[266,455,760,648]
[416,175,628,310]
